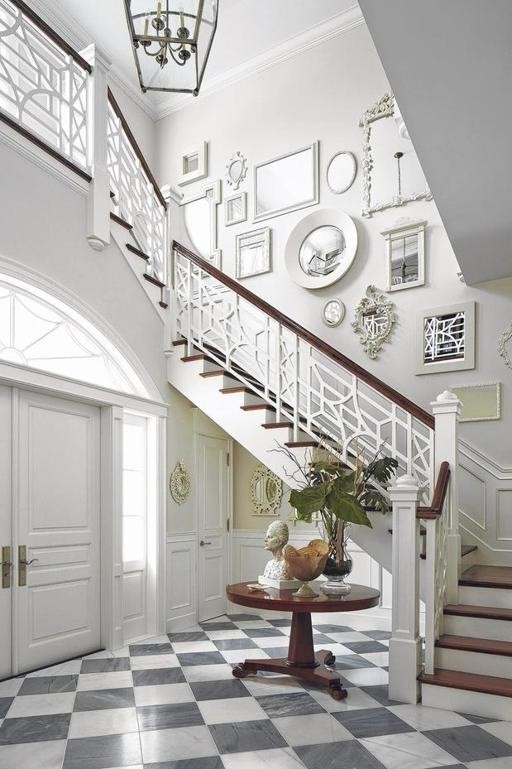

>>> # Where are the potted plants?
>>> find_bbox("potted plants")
[267,434,399,594]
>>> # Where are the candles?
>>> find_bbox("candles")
[182,43,186,51]
[143,18,149,36]
[179,12,185,27]
[161,41,165,55]
[157,1,161,20]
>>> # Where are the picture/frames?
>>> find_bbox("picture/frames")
[450,384,501,423]
[234,226,271,280]
[224,193,247,227]
[380,217,428,293]
[224,150,249,191]
[416,301,476,376]
[177,140,208,185]
[360,93,433,218]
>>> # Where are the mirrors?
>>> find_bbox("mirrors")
[247,464,283,516]
[325,150,358,195]
[350,286,397,360]
[286,206,358,288]
[321,298,346,328]
[250,141,320,224]
